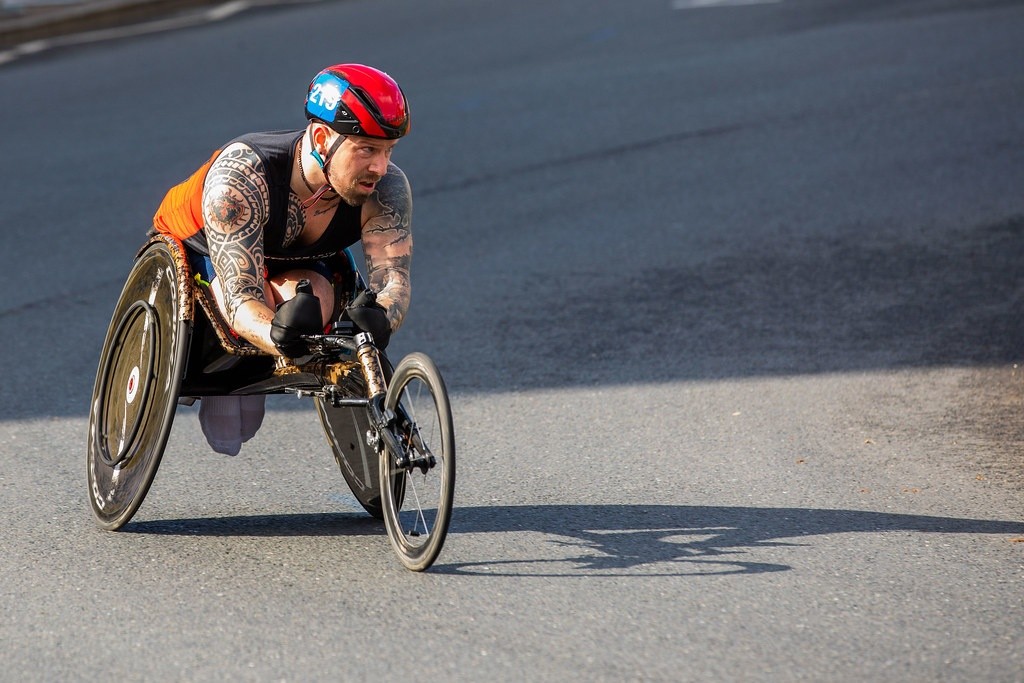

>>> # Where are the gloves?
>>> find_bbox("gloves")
[336,303,392,351]
[269,291,324,359]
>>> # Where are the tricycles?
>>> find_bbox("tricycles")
[86,233,457,572]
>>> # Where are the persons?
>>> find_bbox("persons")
[154,64,411,456]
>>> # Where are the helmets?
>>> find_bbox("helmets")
[303,63,412,139]
